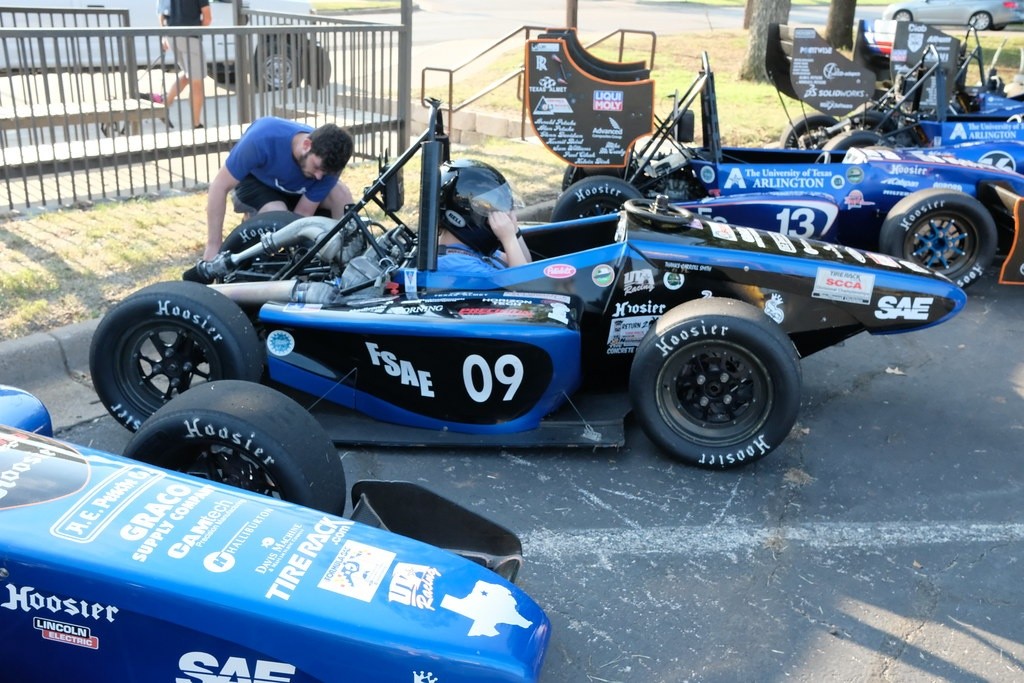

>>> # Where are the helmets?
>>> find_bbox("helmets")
[438,158,514,258]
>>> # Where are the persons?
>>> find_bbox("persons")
[204,116,354,262]
[437,159,533,272]
[156,0,212,129]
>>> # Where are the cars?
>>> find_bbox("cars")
[89,95,967,465]
[523,25,1024,292]
[0,380,551,682]
[1,0,313,91]
[767,25,1024,172]
[882,1,1024,31]
